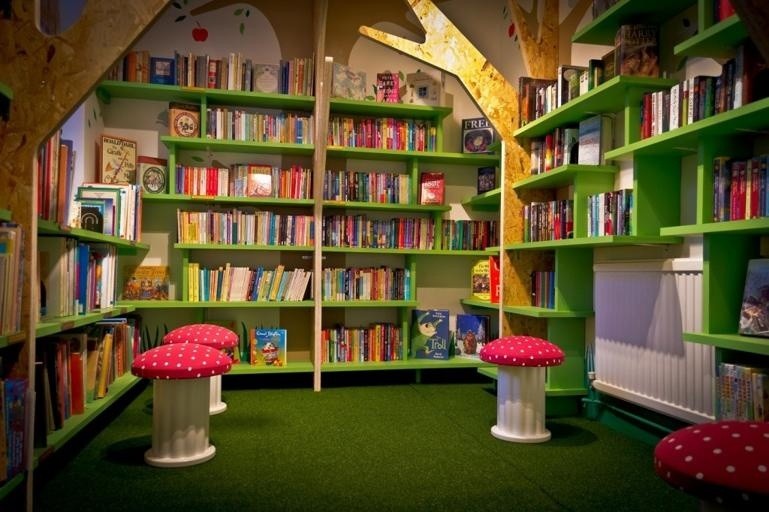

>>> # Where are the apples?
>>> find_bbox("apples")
[508,23,515,36]
[192,22,208,41]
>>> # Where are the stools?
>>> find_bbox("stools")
[131,344,232,468]
[162,324,238,415]
[654,421,768,512]
[479,335,565,443]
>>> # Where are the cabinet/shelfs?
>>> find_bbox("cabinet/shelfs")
[320,57,501,369]
[1,87,31,511]
[81,16,318,380]
[504,1,767,423]
[33,219,150,471]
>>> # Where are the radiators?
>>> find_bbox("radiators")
[591,262,712,423]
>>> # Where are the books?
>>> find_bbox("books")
[588,189,633,237]
[324,265,410,300]
[1,209,24,333]
[713,154,769,222]
[111,51,315,96]
[471,255,500,302]
[518,25,661,128]
[321,321,404,364]
[323,214,496,251]
[176,208,315,245]
[531,114,614,176]
[169,102,314,144]
[327,116,436,152]
[531,271,555,308]
[36,130,167,240]
[38,234,169,316]
[1,317,140,486]
[206,320,287,366]
[462,117,496,194]
[718,362,769,420]
[331,61,445,106]
[640,45,769,140]
[188,262,313,302]
[411,309,492,360]
[176,164,312,199]
[323,170,445,205]
[524,200,573,241]
